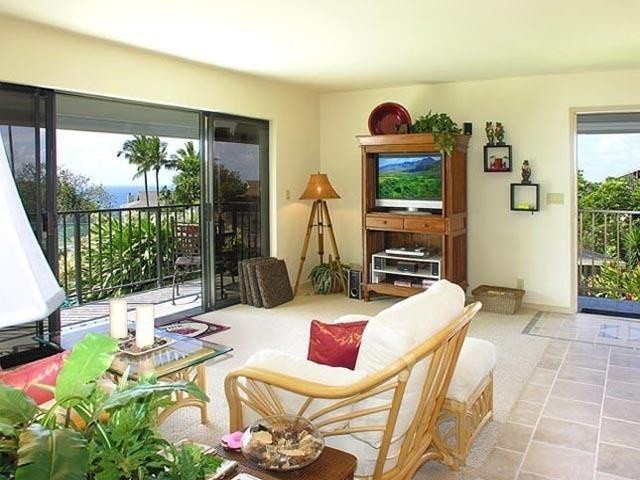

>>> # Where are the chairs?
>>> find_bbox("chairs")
[224,279,482,480]
[170,216,201,307]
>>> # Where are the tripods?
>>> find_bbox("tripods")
[292,201,348,300]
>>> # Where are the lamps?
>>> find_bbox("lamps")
[293,172,349,297]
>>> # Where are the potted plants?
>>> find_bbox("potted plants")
[308,254,351,295]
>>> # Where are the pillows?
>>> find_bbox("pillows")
[307,320,368,370]
[349,277,466,448]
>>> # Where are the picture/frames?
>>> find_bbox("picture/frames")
[510,183,539,211]
[484,145,512,172]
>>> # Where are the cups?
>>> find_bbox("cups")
[109,298,129,339]
[135,305,155,347]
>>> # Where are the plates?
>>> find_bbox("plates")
[116,336,176,355]
[106,327,136,343]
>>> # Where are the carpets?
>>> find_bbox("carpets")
[156,315,231,341]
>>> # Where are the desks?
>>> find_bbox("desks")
[149,424,357,480]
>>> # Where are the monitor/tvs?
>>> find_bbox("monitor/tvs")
[375,155,442,215]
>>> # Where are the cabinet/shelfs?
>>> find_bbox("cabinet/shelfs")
[355,134,467,303]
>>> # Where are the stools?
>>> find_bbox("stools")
[435,335,499,474]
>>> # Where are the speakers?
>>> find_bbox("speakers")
[348,269,359,299]
